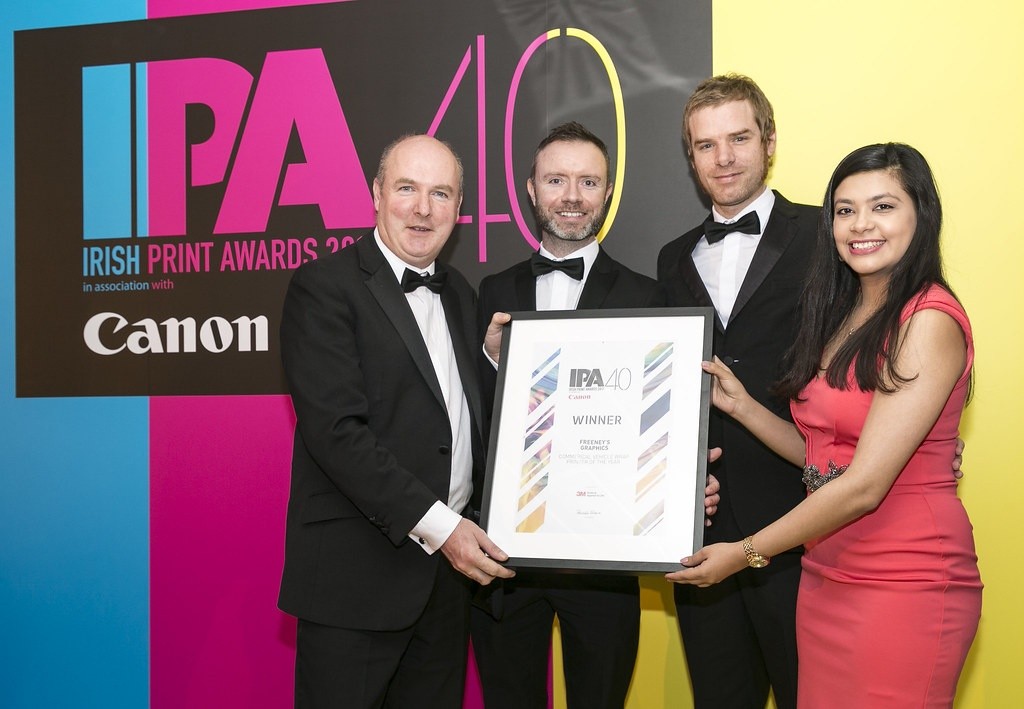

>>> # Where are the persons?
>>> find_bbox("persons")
[277,135,516,709]
[657,75,964,709]
[665,142,985,709]
[473,120,722,709]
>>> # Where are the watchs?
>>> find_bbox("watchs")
[744,536,771,567]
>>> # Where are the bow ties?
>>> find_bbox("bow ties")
[531,252,584,281]
[704,211,761,244]
[400,268,448,295]
